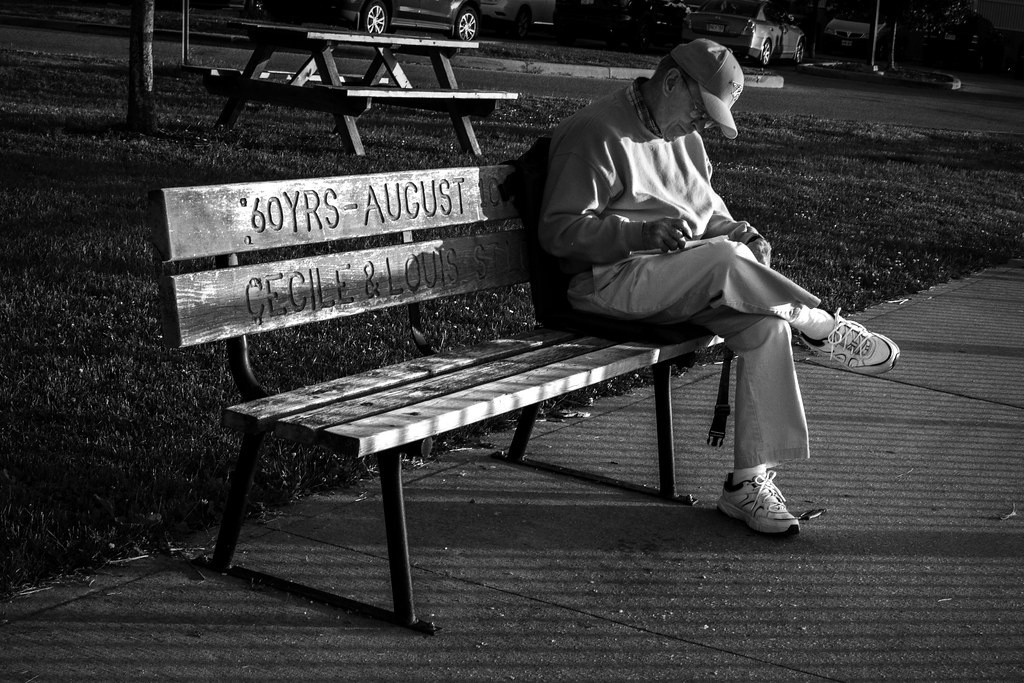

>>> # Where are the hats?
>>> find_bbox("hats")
[670,38,744,140]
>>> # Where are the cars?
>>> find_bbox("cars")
[480,0,1024,55]
[681,0,808,68]
[261,0,482,42]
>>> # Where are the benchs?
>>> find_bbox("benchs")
[181,64,390,132]
[146,164,725,637]
[313,82,518,156]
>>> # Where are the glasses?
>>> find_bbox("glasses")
[681,73,715,130]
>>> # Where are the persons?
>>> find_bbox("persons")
[536,38,901,537]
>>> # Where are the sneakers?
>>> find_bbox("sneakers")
[792,305,900,374]
[717,470,800,535]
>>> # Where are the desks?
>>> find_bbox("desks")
[210,21,482,156]
